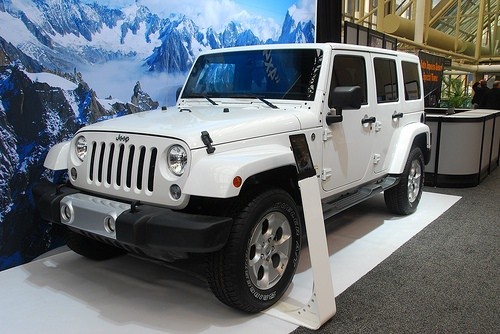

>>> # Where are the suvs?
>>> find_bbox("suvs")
[30,42,432,315]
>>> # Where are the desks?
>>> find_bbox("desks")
[424,106,500,188]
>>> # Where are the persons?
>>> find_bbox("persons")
[472,80,490,109]
[488,81,500,110]
